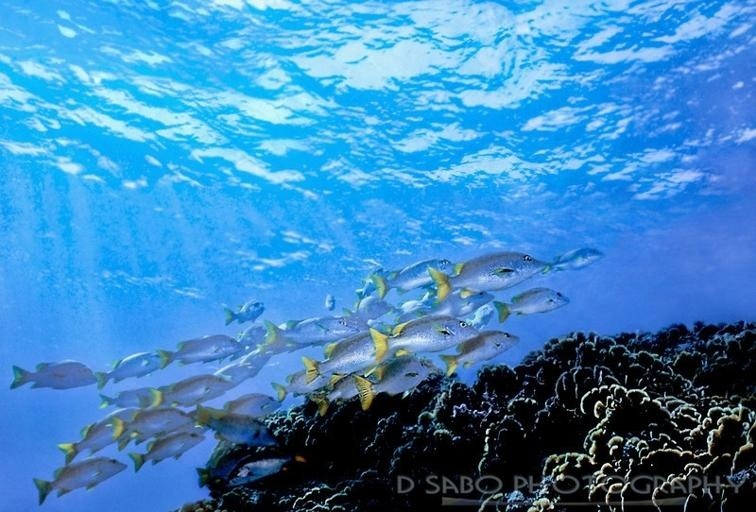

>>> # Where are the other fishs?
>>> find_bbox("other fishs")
[96,259,495,451]
